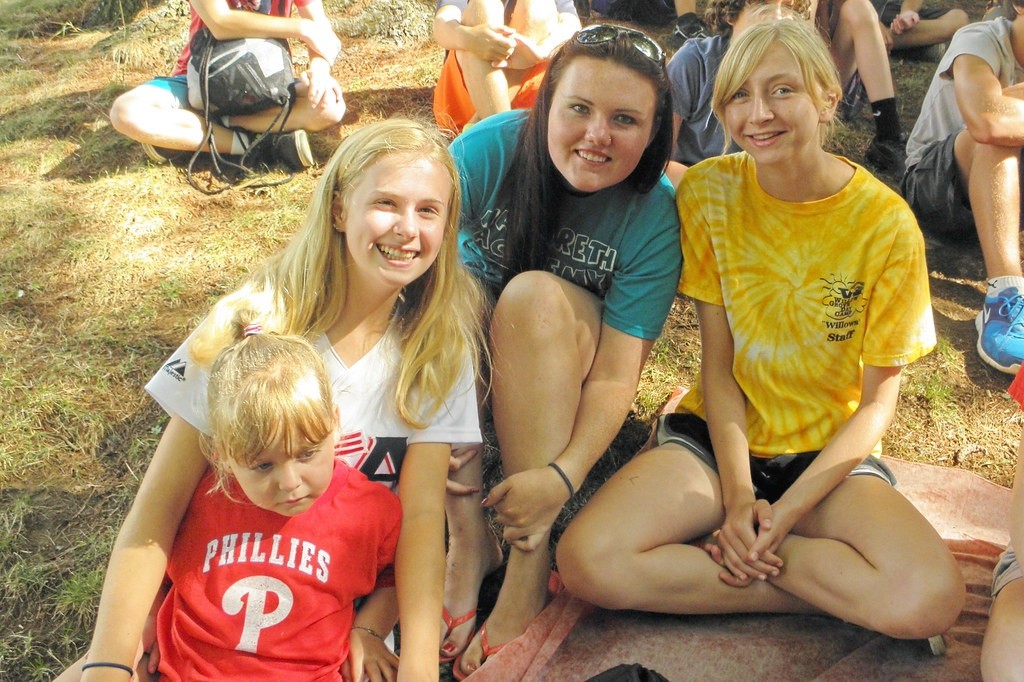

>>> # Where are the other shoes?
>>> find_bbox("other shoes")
[671,23,710,48]
[921,43,945,62]
[866,130,910,182]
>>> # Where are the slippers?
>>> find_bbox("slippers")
[452,570,564,681]
[439,605,476,664]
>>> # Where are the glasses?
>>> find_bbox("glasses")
[577,24,663,62]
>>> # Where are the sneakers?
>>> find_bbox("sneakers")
[142,142,184,164]
[975,287,1024,374]
[249,129,314,168]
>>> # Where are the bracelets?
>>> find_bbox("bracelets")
[82,662,133,677]
[548,463,574,499]
[351,627,385,641]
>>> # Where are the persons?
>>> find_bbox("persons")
[439,24,683,681]
[110,0,346,169]
[900,0,1024,374]
[663,0,811,190]
[83,301,402,681]
[589,0,716,49]
[981,360,1023,682]
[433,0,582,140]
[869,0,970,64]
[810,0,910,187]
[982,0,1004,22]
[557,20,968,658]
[50,119,484,682]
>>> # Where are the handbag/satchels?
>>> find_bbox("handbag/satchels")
[188,28,296,195]
